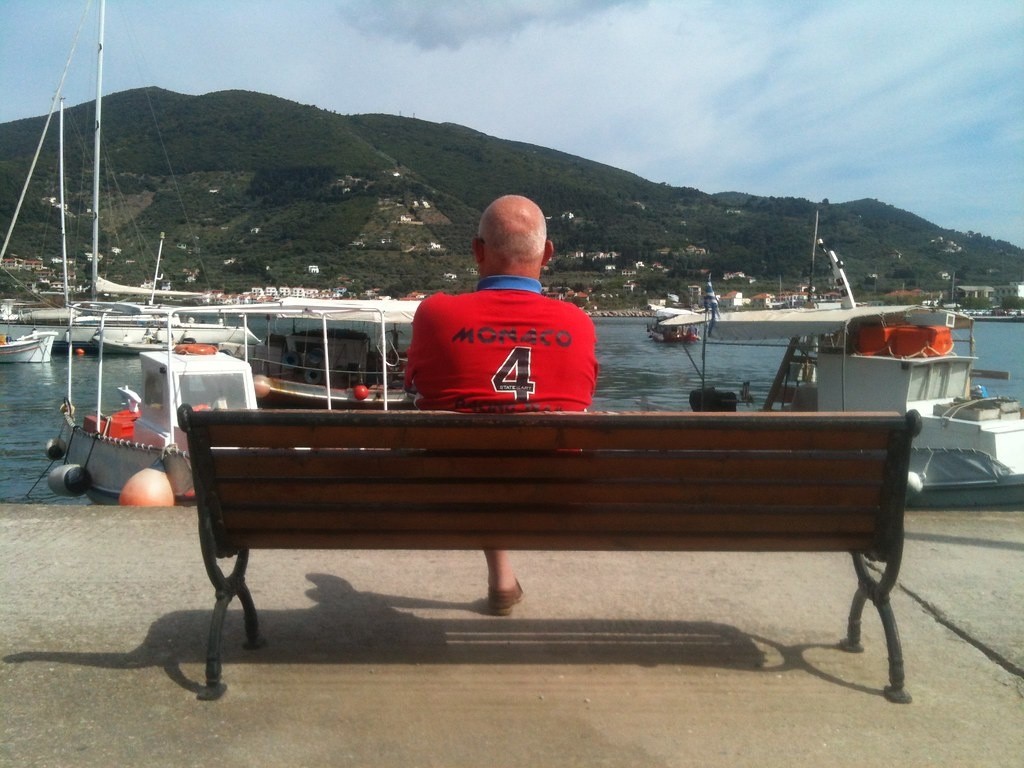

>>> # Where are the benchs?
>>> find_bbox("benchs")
[173,401,922,704]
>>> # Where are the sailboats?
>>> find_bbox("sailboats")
[1,1,266,363]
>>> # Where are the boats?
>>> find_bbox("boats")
[708,211,1024,508]
[646,308,708,342]
[45,299,269,507]
[233,296,430,411]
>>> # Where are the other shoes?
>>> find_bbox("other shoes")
[488,578,524,615]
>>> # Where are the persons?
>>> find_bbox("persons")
[142,328,163,343]
[171,330,189,345]
[405,195,598,615]
[671,325,699,337]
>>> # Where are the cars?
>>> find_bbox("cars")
[961,308,1024,316]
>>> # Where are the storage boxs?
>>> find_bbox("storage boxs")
[851,325,953,358]
[933,397,1021,421]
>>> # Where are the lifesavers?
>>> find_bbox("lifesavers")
[219,349,234,357]
[282,352,301,369]
[175,343,217,355]
[304,366,324,385]
